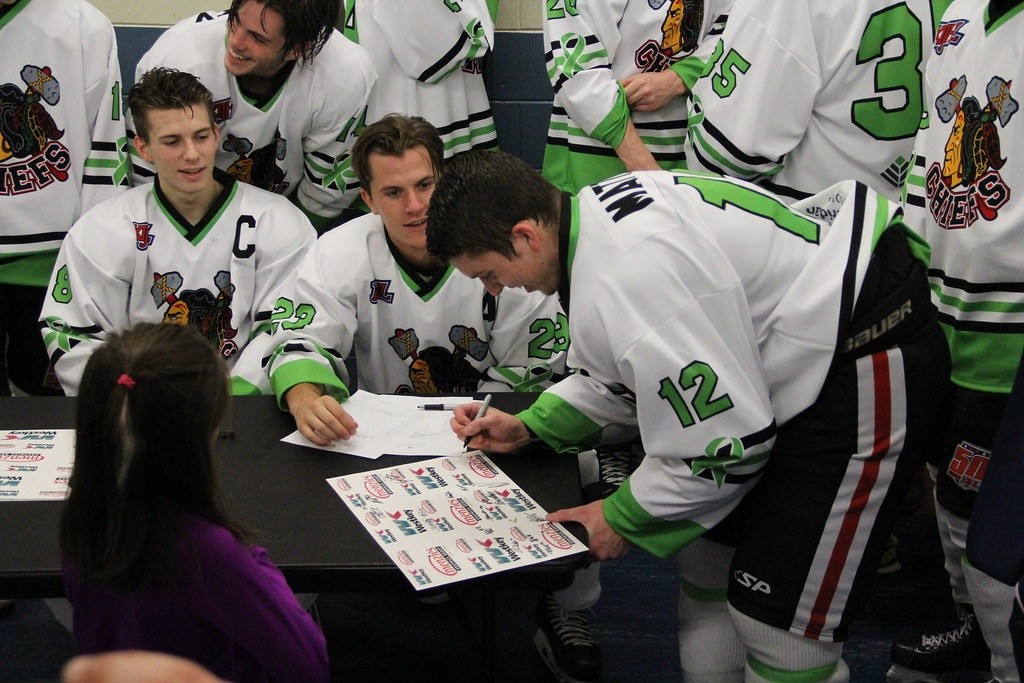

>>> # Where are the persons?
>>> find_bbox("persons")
[38,67,318,397]
[884,0,1024,683]
[133,0,380,235]
[58,320,330,683]
[421,148,953,683]
[262,111,573,447]
[1,0,132,396]
[344,0,952,212]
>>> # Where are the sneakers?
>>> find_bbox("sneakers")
[882,609,1000,683]
[536,591,602,683]
[577,440,645,503]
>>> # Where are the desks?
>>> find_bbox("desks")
[0,391,589,682]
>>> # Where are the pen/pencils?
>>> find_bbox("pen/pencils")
[461,394,493,448]
[415,402,467,412]
